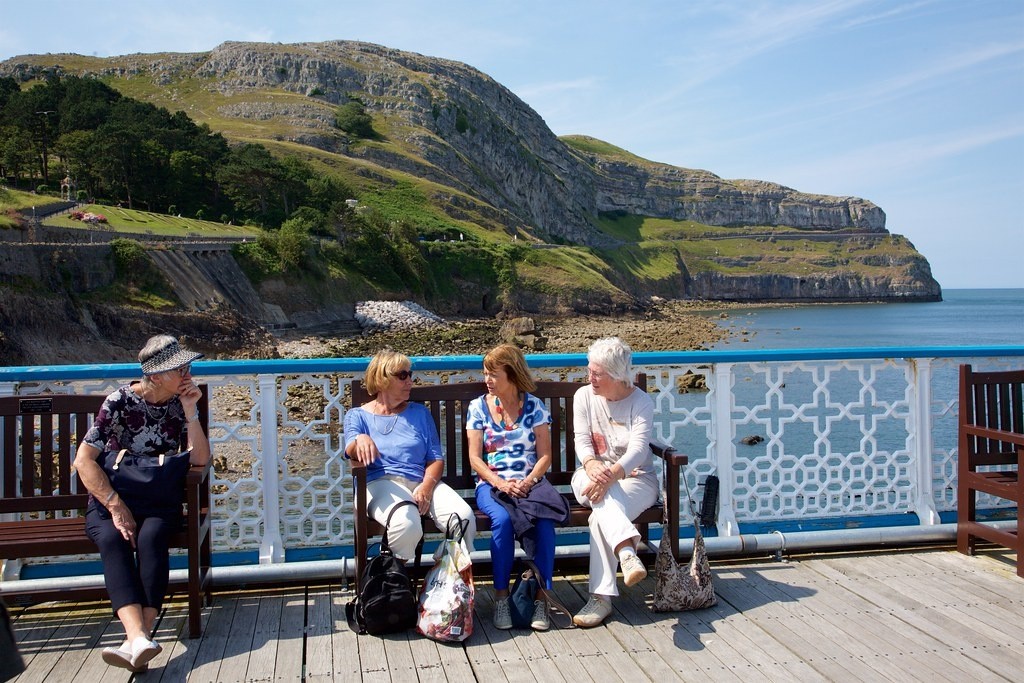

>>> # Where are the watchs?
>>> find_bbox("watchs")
[529,473,538,483]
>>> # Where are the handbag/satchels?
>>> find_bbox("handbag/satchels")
[416,512,475,644]
[655,447,719,610]
[512,560,573,630]
[93,446,194,519]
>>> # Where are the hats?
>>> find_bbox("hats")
[142,342,205,375]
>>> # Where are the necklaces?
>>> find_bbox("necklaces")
[140,382,169,421]
[495,395,523,431]
[373,401,398,435]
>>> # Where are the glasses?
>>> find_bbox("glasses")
[174,365,192,378]
[583,368,605,378]
[395,370,415,381]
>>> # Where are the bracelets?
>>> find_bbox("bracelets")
[103,490,115,507]
[188,409,200,422]
[423,475,437,485]
[583,459,597,471]
[355,435,358,444]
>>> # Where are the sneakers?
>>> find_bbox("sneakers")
[573,593,613,628]
[494,593,514,629]
[531,599,550,630]
[620,553,646,587]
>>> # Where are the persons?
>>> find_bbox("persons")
[466,343,554,631]
[571,337,658,626]
[344,349,476,570]
[73,335,210,671]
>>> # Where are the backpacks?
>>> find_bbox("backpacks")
[346,500,425,636]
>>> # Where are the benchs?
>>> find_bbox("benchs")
[956,364,1024,578]
[350,372,689,596]
[0,381,214,640]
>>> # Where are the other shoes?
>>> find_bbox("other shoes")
[102,647,149,673]
[131,640,163,667]
